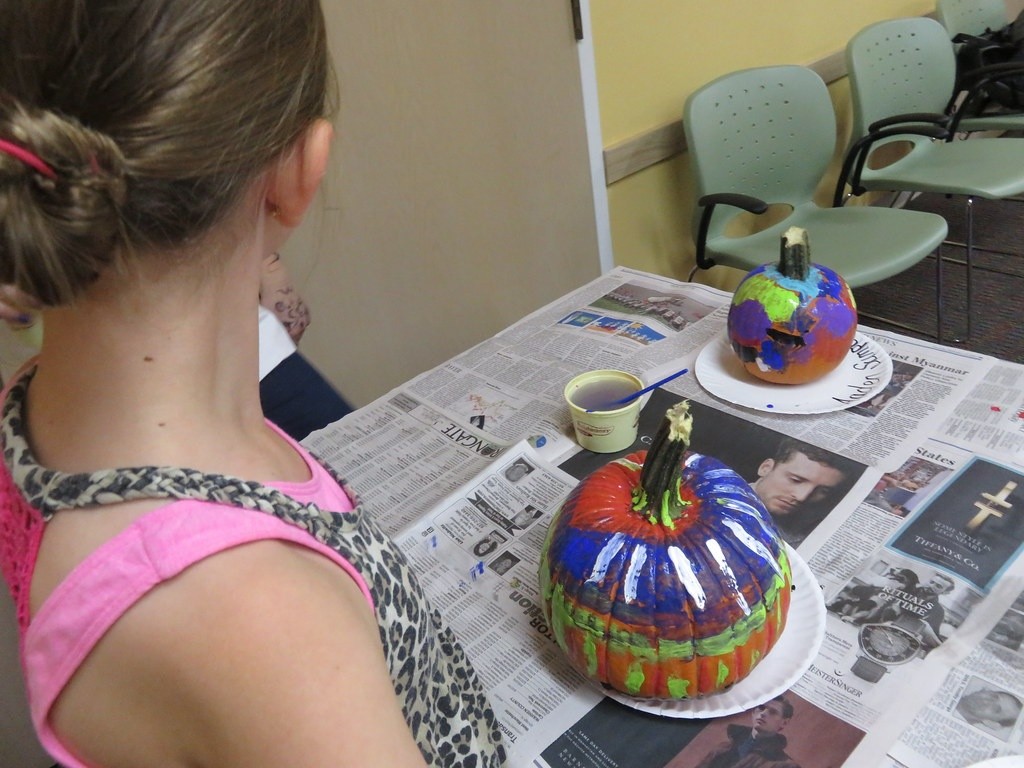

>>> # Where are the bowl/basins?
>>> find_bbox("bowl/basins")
[564,369,645,454]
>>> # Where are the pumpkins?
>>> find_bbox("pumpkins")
[537,399,794,702]
[726,225,859,386]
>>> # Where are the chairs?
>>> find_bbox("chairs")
[832,16,1024,343]
[937,2,1024,138]
[682,63,950,347]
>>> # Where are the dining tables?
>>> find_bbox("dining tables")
[274,266,1024,768]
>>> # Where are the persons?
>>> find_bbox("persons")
[1,1,507,768]
[695,696,802,768]
[956,690,1023,727]
[824,569,957,646]
[746,437,858,547]
[473,458,544,558]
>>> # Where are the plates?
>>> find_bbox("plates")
[585,539,825,718]
[694,326,892,415]
